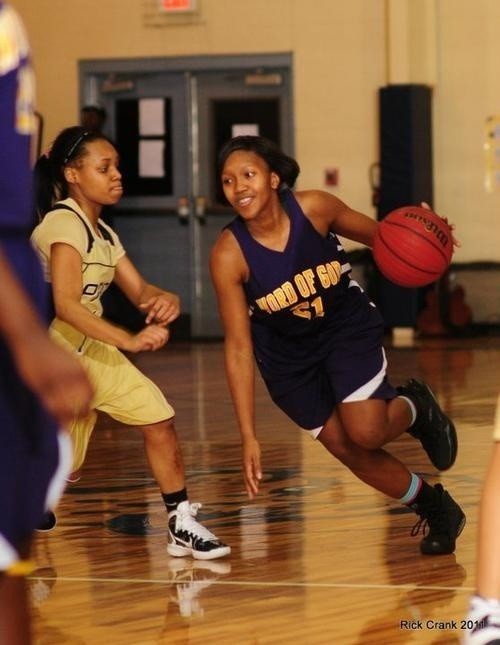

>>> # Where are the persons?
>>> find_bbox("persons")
[77,101,121,233]
[33,104,53,220]
[461,386,499,644]
[1,0,101,644]
[208,136,466,557]
[30,126,229,561]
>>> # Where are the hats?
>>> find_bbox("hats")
[214,133,302,190]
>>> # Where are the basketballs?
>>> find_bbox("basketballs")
[373,204,453,288]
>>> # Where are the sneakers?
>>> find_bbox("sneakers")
[165,499,233,561]
[394,376,459,472]
[407,483,468,555]
